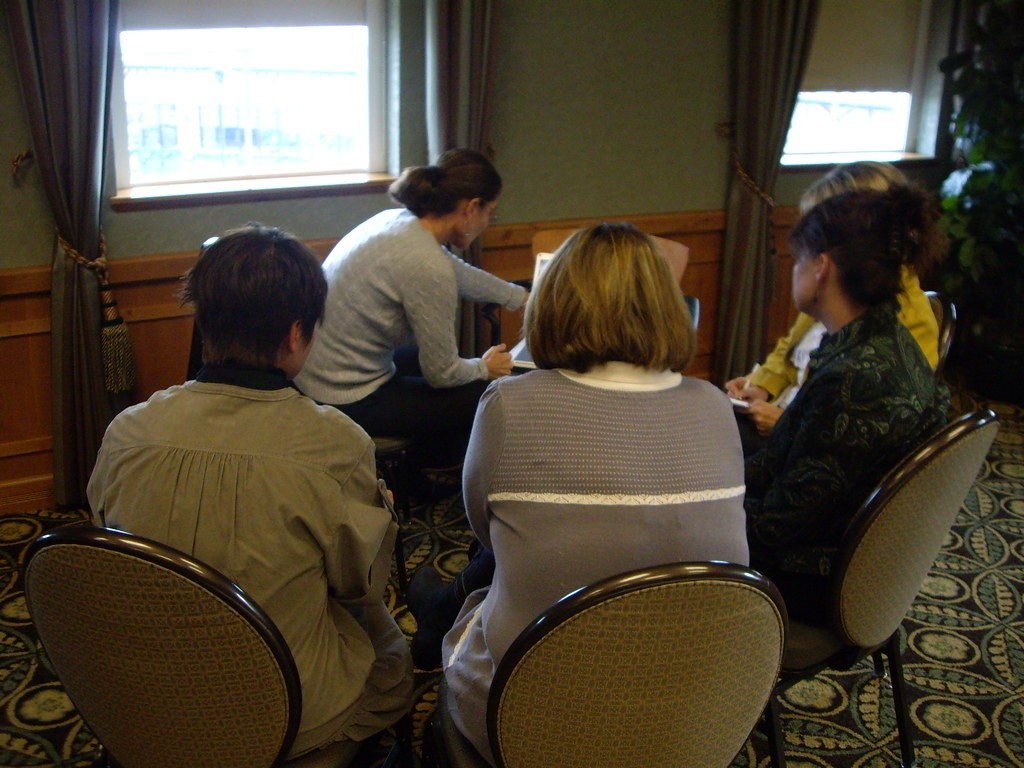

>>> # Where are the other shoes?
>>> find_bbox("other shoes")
[395,472,444,505]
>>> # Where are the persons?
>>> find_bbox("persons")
[718,160,951,630]
[435,219,754,768]
[287,147,532,507]
[86,227,419,768]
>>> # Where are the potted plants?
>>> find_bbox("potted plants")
[932,0,1023,407]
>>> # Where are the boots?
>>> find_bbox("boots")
[408,564,461,671]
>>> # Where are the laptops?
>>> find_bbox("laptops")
[509,252,555,369]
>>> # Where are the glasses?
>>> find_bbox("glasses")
[482,199,501,222]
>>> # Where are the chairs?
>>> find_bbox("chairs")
[20,527,415,768]
[482,229,700,347]
[926,292,956,370]
[441,558,787,767]
[774,409,1000,768]
